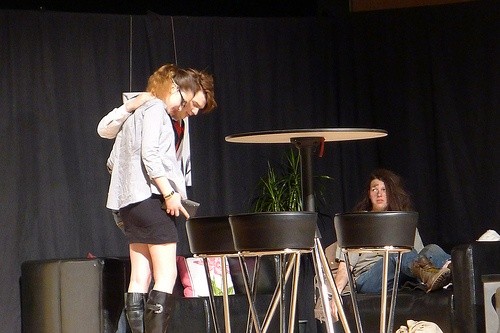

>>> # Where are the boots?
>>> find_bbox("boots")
[411,254,453,293]
[124,289,174,333]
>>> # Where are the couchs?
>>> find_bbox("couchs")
[298,246,494,333]
[21,257,131,333]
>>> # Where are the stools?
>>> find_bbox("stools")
[334,211,418,333]
[185,211,318,333]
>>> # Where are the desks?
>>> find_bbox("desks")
[224,128,392,333]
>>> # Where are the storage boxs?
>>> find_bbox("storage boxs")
[481,274,500,333]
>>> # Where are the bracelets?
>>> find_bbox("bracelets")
[164,188,176,199]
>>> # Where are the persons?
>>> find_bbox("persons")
[329,166,453,323]
[97,62,216,333]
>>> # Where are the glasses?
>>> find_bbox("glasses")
[178,89,187,107]
